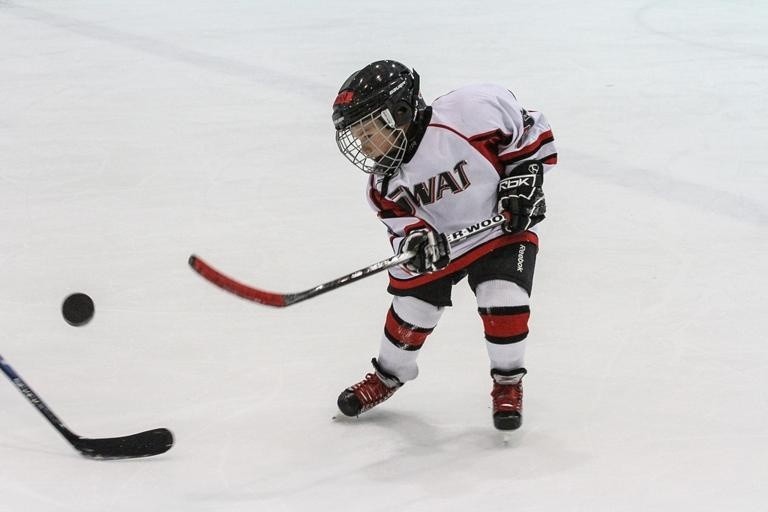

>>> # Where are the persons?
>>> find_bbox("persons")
[329,57,560,432]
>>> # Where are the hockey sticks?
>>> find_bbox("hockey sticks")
[189,210,510,307]
[0,359,174,459]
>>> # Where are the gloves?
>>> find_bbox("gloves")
[403,229,451,275]
[496,159,547,236]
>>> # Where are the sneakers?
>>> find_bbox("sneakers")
[337,357,405,417]
[490,367,528,430]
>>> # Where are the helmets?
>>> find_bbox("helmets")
[330,59,426,181]
